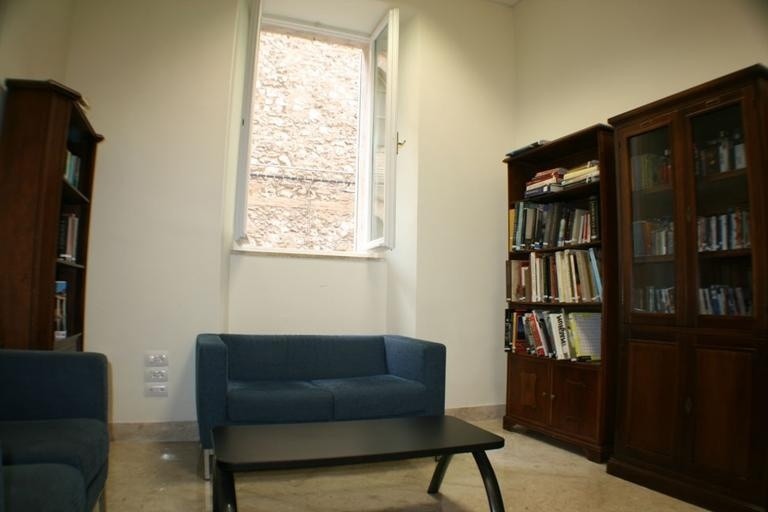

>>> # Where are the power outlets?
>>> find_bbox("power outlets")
[144,351,169,397]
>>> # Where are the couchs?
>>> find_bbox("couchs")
[0,350,111,512]
[197,335,452,454]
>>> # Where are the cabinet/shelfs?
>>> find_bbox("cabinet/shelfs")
[503,124,616,464]
[0,75,104,349]
[606,64,768,511]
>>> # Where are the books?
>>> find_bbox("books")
[54,147,83,339]
[507,160,603,364]
[630,131,754,316]
